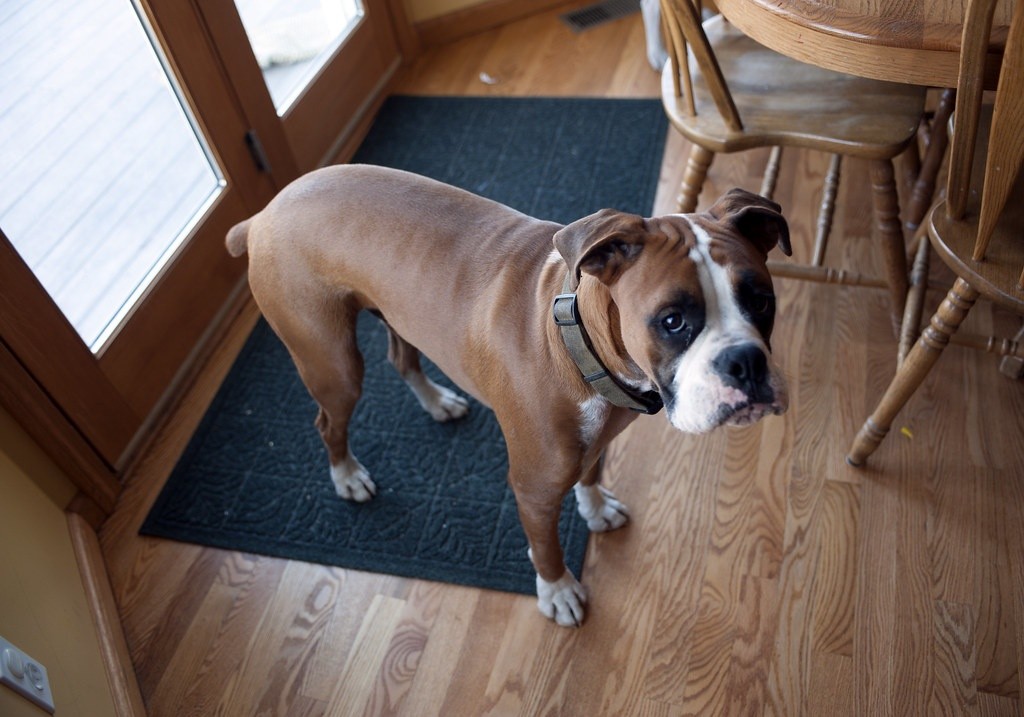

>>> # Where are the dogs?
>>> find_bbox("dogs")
[225,166,794,627]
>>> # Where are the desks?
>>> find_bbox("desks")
[711,0,1024,231]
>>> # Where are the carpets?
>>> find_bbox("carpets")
[132,98,674,598]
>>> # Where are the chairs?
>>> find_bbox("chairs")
[847,0,1024,467]
[656,0,928,350]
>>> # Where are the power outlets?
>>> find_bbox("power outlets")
[0,637,55,713]
